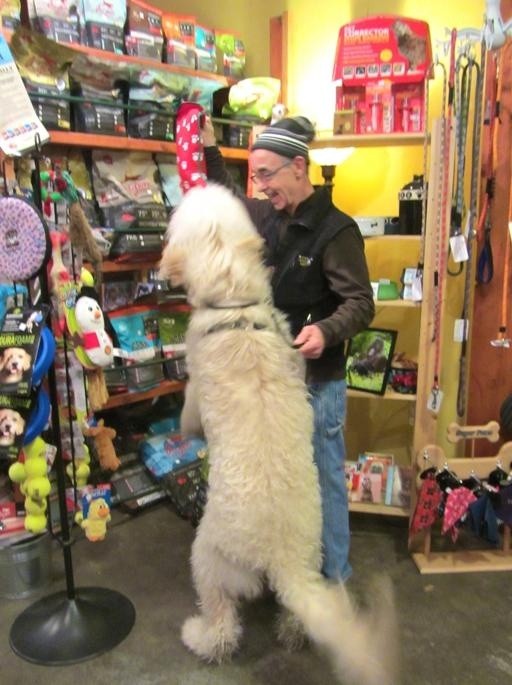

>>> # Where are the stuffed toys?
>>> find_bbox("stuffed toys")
[80,497,112,543]
[83,419,121,471]
[0,149,124,543]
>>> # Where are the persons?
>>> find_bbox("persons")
[197,103,377,610]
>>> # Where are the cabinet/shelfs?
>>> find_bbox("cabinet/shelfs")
[41,117,249,532]
[249,123,446,531]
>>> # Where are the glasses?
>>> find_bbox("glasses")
[249,160,295,186]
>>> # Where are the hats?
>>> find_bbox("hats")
[250,113,320,168]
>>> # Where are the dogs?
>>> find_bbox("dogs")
[155,181,395,685]
